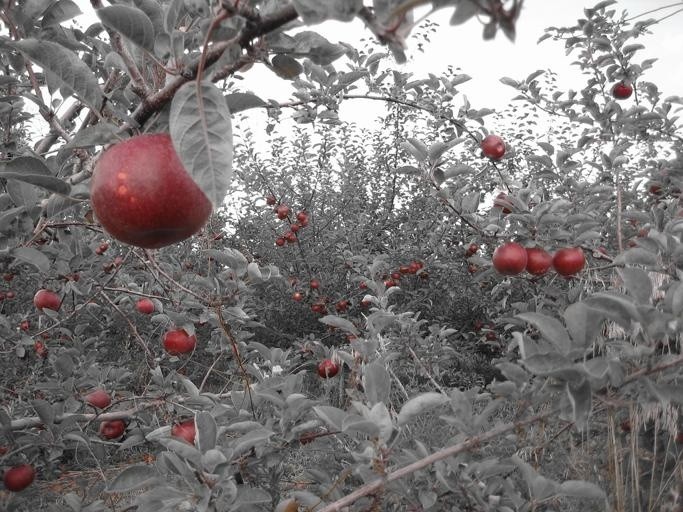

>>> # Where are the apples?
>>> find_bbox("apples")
[0,84,683,491]
